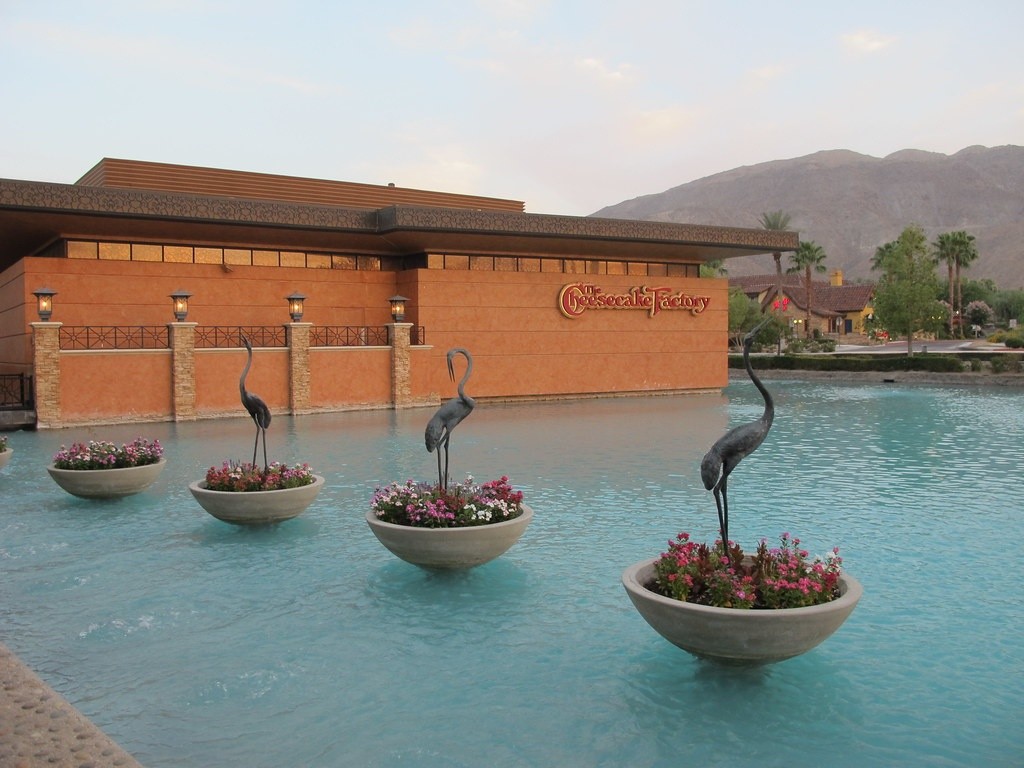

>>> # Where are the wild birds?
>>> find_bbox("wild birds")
[701,315,776,573]
[425,348,475,495]
[236,331,272,476]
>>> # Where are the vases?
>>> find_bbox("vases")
[189,474,326,527]
[364,502,534,573]
[46,458,166,501]
[622,552,865,668]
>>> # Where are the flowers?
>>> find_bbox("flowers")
[652,531,843,609]
[52,436,165,470]
[368,472,522,527]
[203,459,317,492]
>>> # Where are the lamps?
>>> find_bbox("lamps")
[30,286,58,321]
[167,288,194,322]
[283,290,309,323]
[387,295,411,322]
[222,262,236,276]
